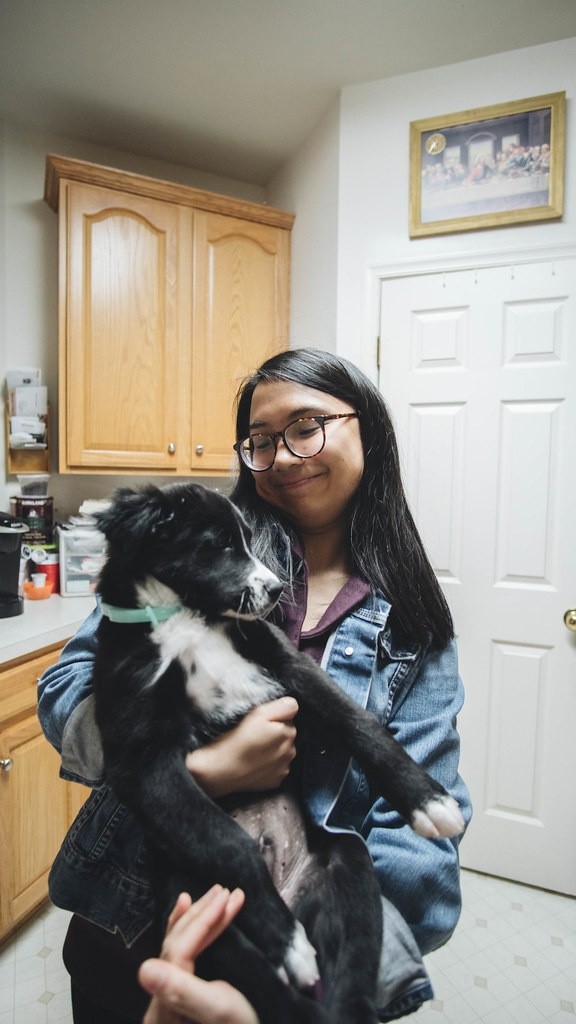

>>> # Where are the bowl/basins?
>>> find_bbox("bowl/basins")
[17,473,51,497]
[24,583,54,600]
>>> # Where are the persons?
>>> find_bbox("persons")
[39,343,480,1024]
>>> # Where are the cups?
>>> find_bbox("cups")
[30,574,48,589]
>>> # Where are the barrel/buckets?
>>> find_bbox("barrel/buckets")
[8,495,53,545]
[35,563,59,593]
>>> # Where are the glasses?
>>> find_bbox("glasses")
[232,412,361,471]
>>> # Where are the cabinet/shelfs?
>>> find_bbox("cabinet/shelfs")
[1,650,102,942]
[43,150,296,475]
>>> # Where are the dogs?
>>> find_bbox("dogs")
[90,483,466,1024]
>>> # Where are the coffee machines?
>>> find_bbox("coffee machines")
[0,510,30,620]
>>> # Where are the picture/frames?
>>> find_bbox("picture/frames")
[408,91,565,239]
